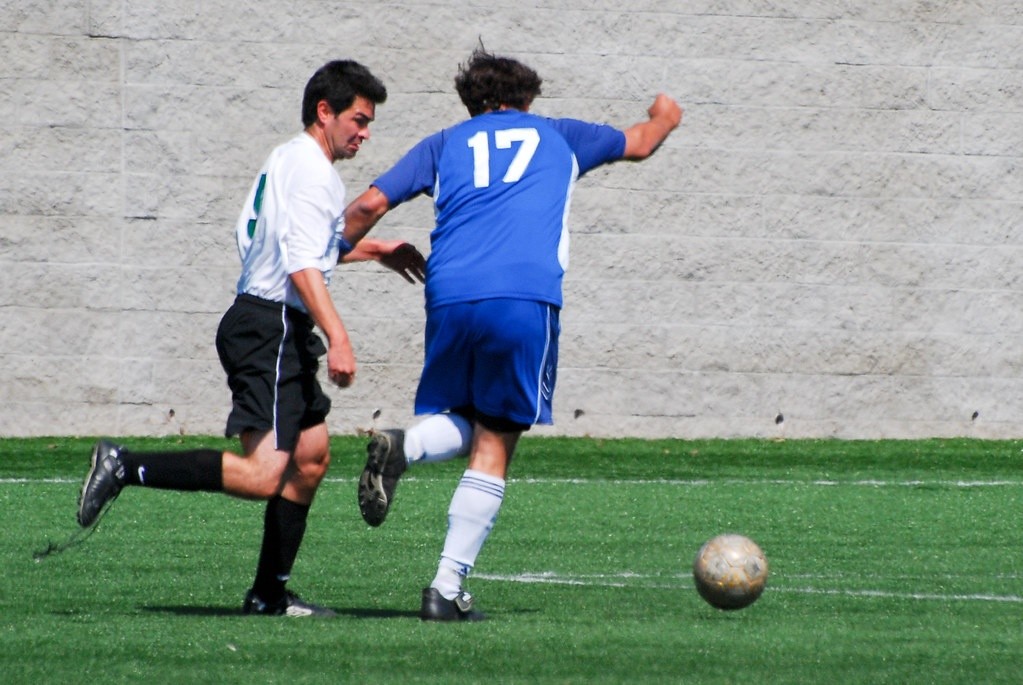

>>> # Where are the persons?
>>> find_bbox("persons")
[341,51,683,621]
[76,59,426,618]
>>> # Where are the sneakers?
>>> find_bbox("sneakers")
[421,587,485,623]
[358,428,407,527]
[243,588,335,618]
[77,439,128,529]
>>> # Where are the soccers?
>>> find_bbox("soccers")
[691,532,770,610]
